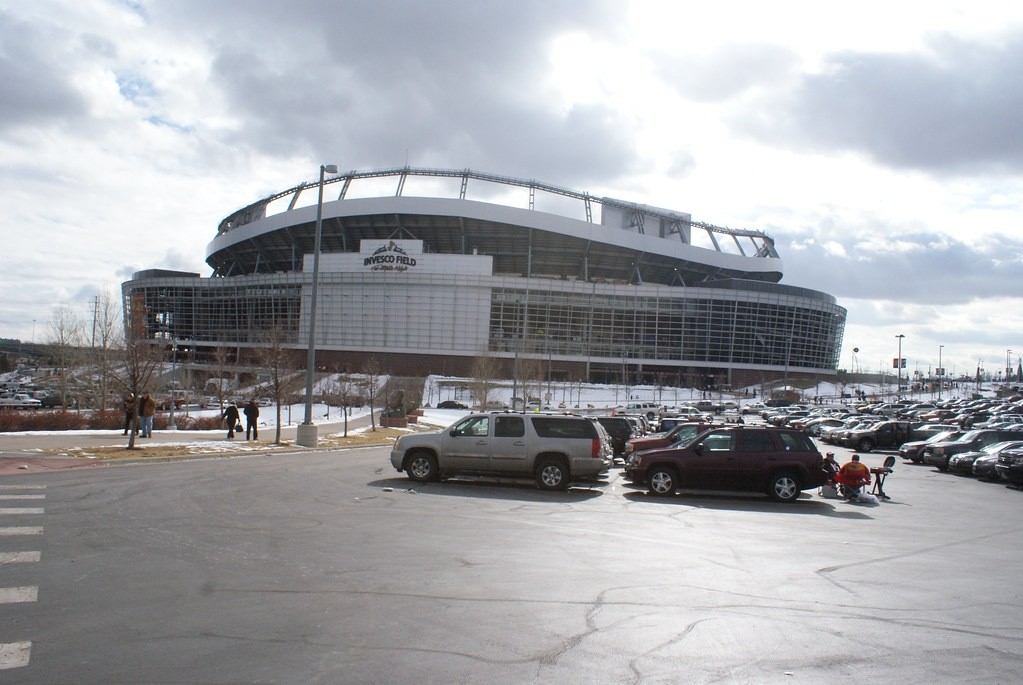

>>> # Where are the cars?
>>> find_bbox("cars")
[437,401,468,409]
[481,399,510,409]
[597,391,1023,502]
[0,368,272,411]
[943,384,948,388]
[522,400,554,411]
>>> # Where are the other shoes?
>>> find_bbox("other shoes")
[148,433,151,438]
[140,435,147,438]
[122,434,128,435]
[136,431,139,434]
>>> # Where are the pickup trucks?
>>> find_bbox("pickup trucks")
[840,420,941,453]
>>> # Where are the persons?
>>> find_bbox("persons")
[221,400,240,439]
[703,391,706,399]
[244,399,259,440]
[709,393,712,399]
[753,388,756,398]
[140,391,156,438]
[831,455,872,499]
[822,452,840,486]
[725,416,744,428]
[855,389,866,401]
[630,395,633,400]
[503,406,510,413]
[122,393,139,435]
[470,410,474,415]
[814,396,823,405]
[900,381,958,393]
[745,387,748,396]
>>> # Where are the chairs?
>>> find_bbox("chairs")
[844,462,865,502]
[818,459,837,498]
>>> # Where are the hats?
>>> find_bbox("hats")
[827,452,835,456]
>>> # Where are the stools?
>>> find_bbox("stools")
[866,455,895,499]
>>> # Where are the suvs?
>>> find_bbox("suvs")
[622,426,830,503]
[390,410,612,491]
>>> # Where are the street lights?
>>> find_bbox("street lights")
[938,345,943,399]
[896,335,905,400]
[1007,349,1012,389]
[784,339,792,390]
[305,164,337,425]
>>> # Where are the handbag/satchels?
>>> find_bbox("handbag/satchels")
[236,421,243,432]
